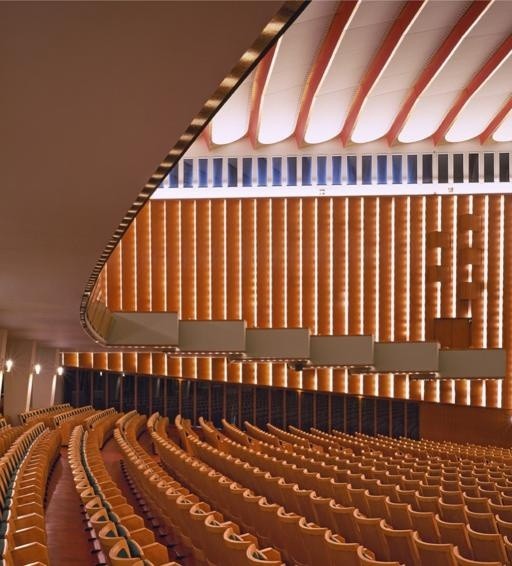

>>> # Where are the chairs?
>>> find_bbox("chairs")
[0,401,512,565]
